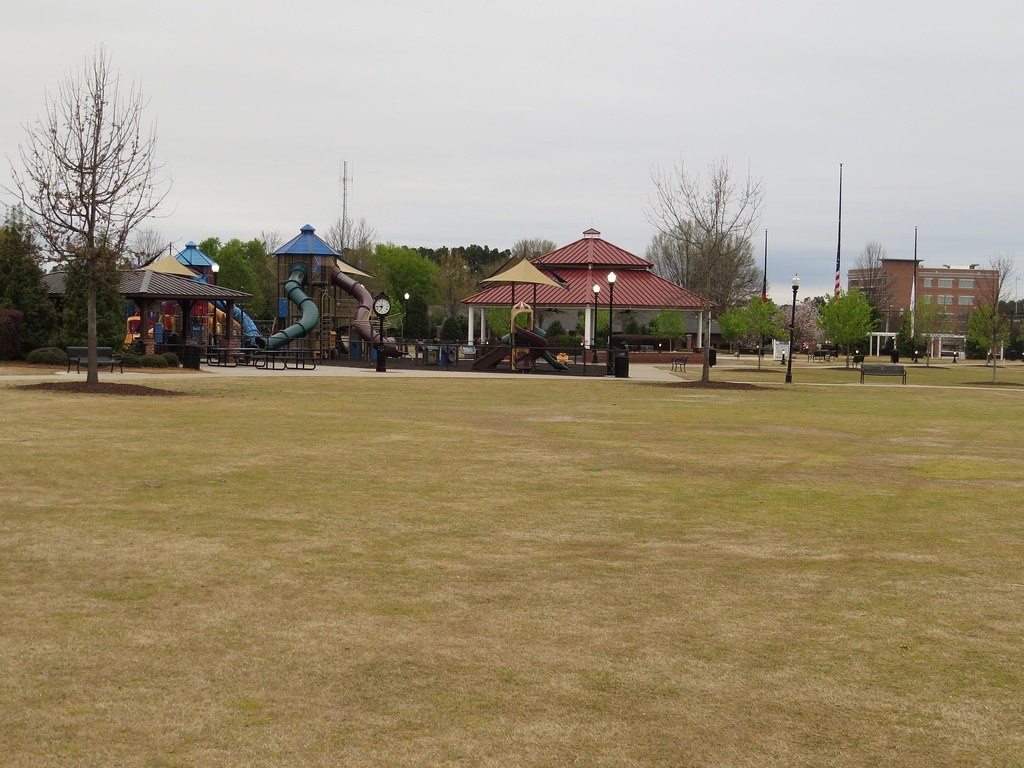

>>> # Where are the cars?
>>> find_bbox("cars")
[941,351,959,359]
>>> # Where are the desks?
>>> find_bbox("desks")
[212,347,259,351]
[255,350,312,355]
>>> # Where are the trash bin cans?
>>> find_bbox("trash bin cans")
[441,342,456,366]
[750,349,753,355]
[734,350,739,357]
[423,346,439,365]
[614,356,630,377]
[182,344,203,370]
[709,349,717,367]
[890,349,899,362]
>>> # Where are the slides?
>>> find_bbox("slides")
[260,262,320,355]
[513,324,549,370]
[534,325,569,370]
[330,265,404,358]
[472,343,510,370]
[192,275,261,353]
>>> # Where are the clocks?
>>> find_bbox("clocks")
[374,299,390,314]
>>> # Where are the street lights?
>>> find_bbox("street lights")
[785,273,801,382]
[606,271,617,375]
[404,293,410,352]
[1016,276,1020,313]
[212,263,220,345]
[592,284,600,363]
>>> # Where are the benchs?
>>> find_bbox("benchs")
[670,355,688,373]
[273,356,320,370]
[859,363,907,385]
[203,353,245,368]
[67,346,123,374]
[808,349,828,362]
[252,355,296,369]
[849,355,864,368]
